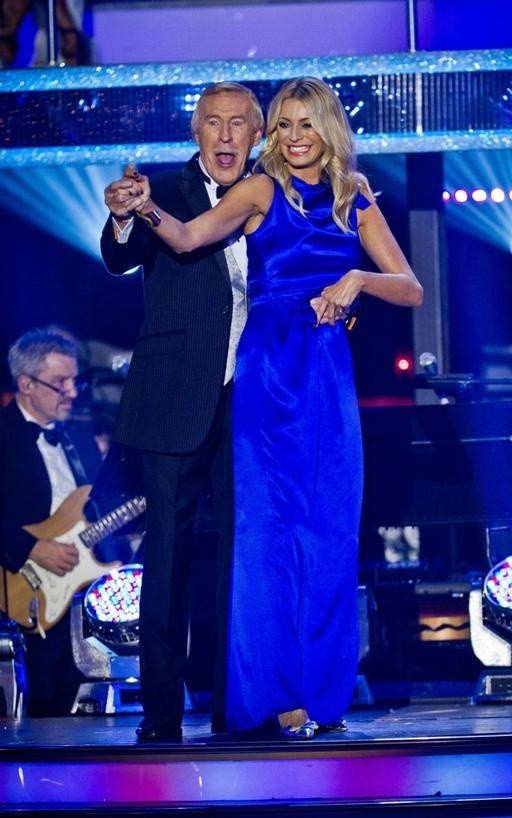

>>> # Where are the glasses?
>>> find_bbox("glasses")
[28,374,88,400]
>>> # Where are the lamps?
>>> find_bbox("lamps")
[70,562,194,714]
[470,554,512,704]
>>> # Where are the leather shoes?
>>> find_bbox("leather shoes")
[135,718,181,736]
[274,709,319,739]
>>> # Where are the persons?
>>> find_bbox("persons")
[100,82,363,743]
[127,75,426,739]
[2,329,132,719]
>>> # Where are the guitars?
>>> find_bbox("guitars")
[1,482,149,633]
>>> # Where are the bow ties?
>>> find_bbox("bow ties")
[28,421,61,447]
[216,185,229,198]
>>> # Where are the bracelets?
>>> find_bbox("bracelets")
[141,209,164,227]
